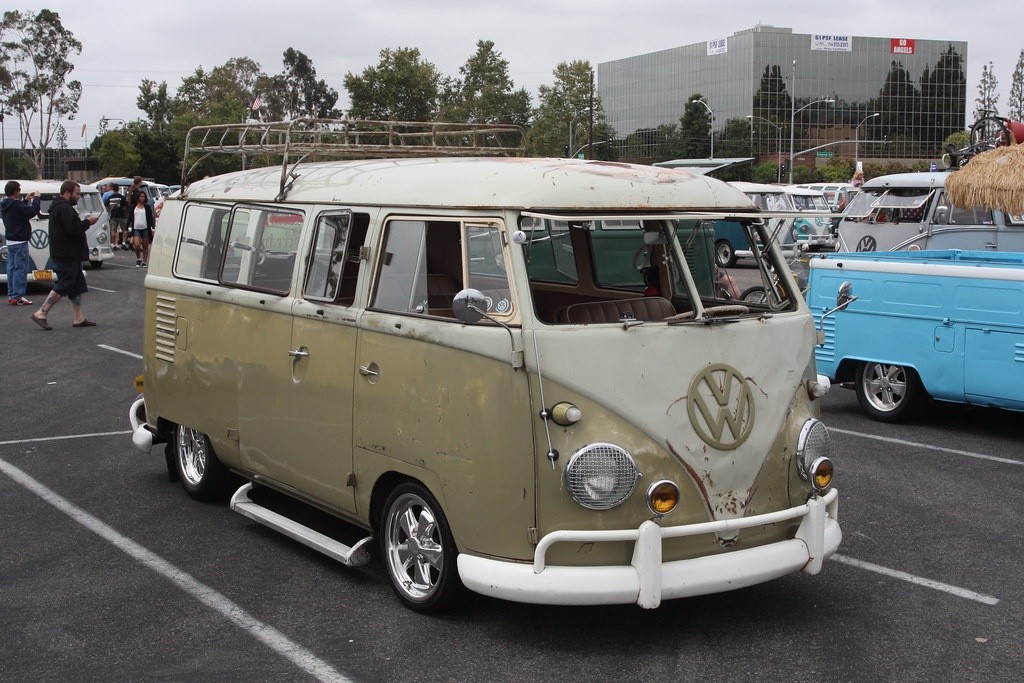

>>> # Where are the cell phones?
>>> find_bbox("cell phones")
[98,210,104,218]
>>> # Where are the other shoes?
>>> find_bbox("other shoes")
[136,260,141,268]
[128,243,135,251]
[121,245,129,251]
[142,263,147,267]
[113,246,117,251]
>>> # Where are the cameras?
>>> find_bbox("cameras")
[30,193,35,196]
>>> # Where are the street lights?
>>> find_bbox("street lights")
[568,108,607,158]
[855,113,880,171]
[788,59,835,187]
[692,100,714,159]
[746,115,787,184]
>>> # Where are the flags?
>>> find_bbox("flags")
[252,94,262,110]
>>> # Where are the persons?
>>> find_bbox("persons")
[124,190,155,267]
[775,164,785,183]
[852,169,863,187]
[101,176,144,252]
[0,181,41,305]
[31,181,99,331]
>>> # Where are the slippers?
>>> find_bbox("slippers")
[73,318,96,327]
[30,315,52,330]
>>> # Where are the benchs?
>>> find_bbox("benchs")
[252,273,677,325]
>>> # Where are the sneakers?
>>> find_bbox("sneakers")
[8,297,32,306]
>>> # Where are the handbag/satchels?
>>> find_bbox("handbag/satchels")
[109,210,112,219]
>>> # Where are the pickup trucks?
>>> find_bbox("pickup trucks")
[804,248,1024,425]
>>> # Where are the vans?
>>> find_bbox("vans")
[521,167,1024,297]
[129,121,859,617]
[0,174,186,285]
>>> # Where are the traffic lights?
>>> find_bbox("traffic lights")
[563,144,569,155]
[884,135,892,150]
[606,136,613,150]
